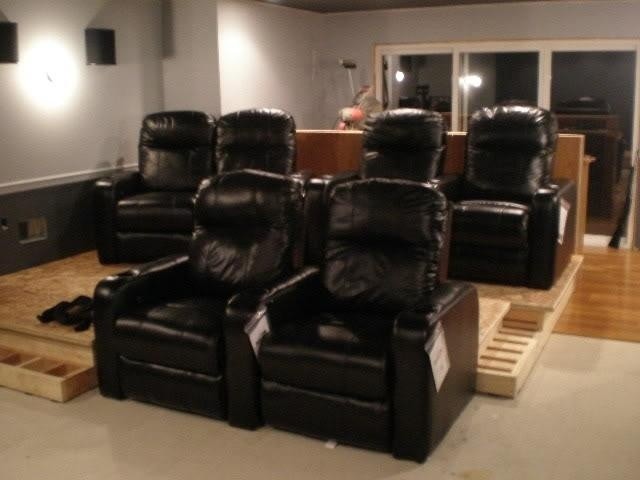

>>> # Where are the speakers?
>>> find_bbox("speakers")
[0,22,18,64]
[85,28,116,64]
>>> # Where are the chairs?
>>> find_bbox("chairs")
[213,106,311,180]
[237,176,480,463]
[434,97,579,292]
[89,107,215,261]
[307,105,448,278]
[92,169,239,429]
